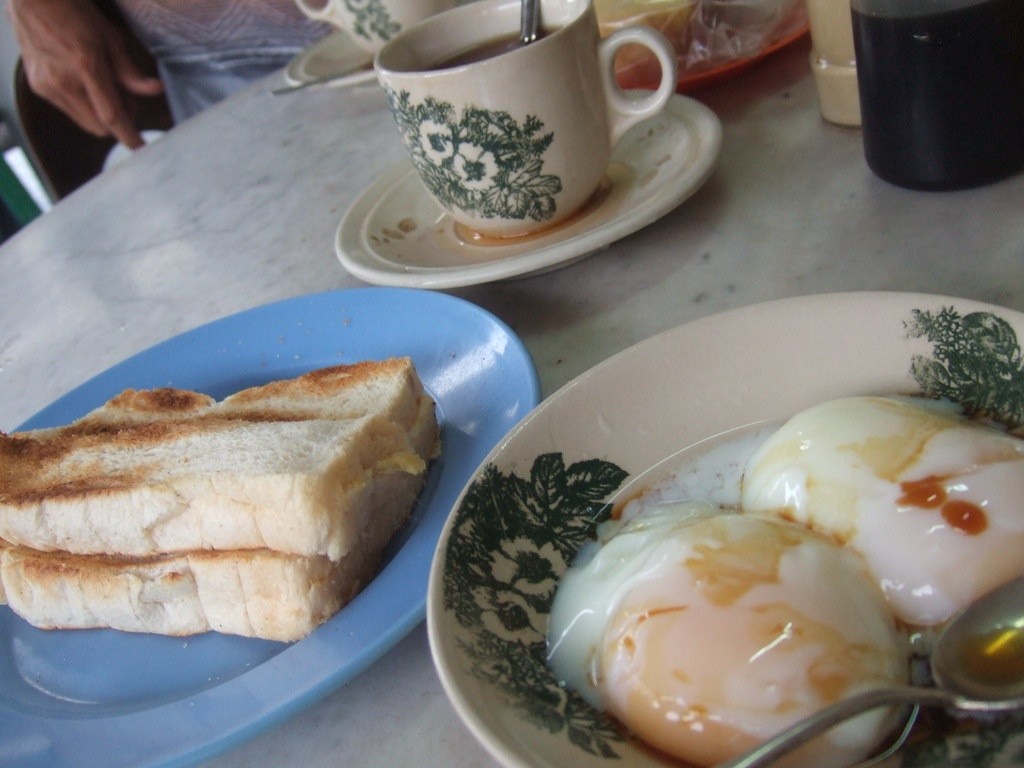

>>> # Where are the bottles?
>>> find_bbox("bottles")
[806,0,862,127]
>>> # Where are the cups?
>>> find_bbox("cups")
[848,0,1024,189]
[374,0,680,240]
[294,0,460,55]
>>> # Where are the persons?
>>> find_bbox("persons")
[7,0,341,151]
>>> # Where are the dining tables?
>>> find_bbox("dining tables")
[0,35,1024,768]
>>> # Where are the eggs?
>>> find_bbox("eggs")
[545,497,913,768]
[735,396,1024,635]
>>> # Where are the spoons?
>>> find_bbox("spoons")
[728,574,1024,768]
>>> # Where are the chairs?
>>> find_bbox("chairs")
[3,31,173,215]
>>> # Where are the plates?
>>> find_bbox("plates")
[333,88,723,288]
[0,284,545,767]
[424,290,1024,768]
[614,0,810,91]
[283,29,372,87]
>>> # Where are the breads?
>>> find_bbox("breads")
[0,355,440,645]
[592,0,698,70]
